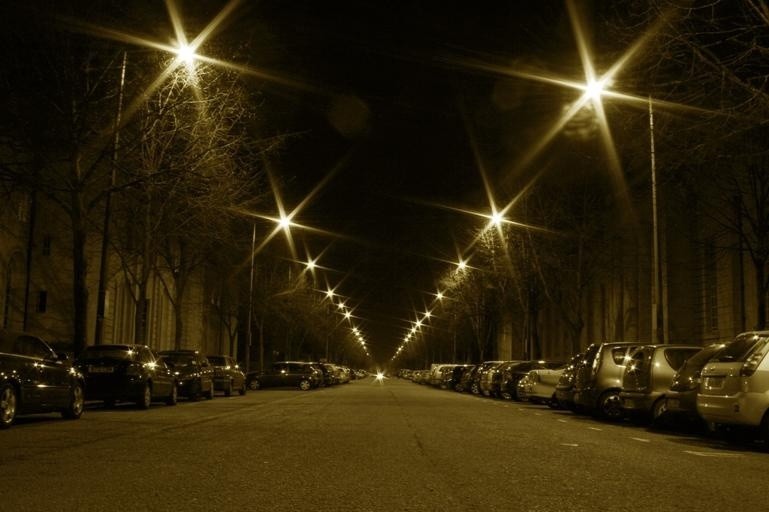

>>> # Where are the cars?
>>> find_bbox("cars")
[157,349,216,404]
[391,339,670,427]
[695,329,769,450]
[671,338,736,433]
[299,358,371,388]
[0,328,88,430]
[245,360,324,392]
[206,354,248,399]
[617,342,704,429]
[68,341,180,410]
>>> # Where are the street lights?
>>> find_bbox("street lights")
[565,70,662,345]
[93,25,209,345]
